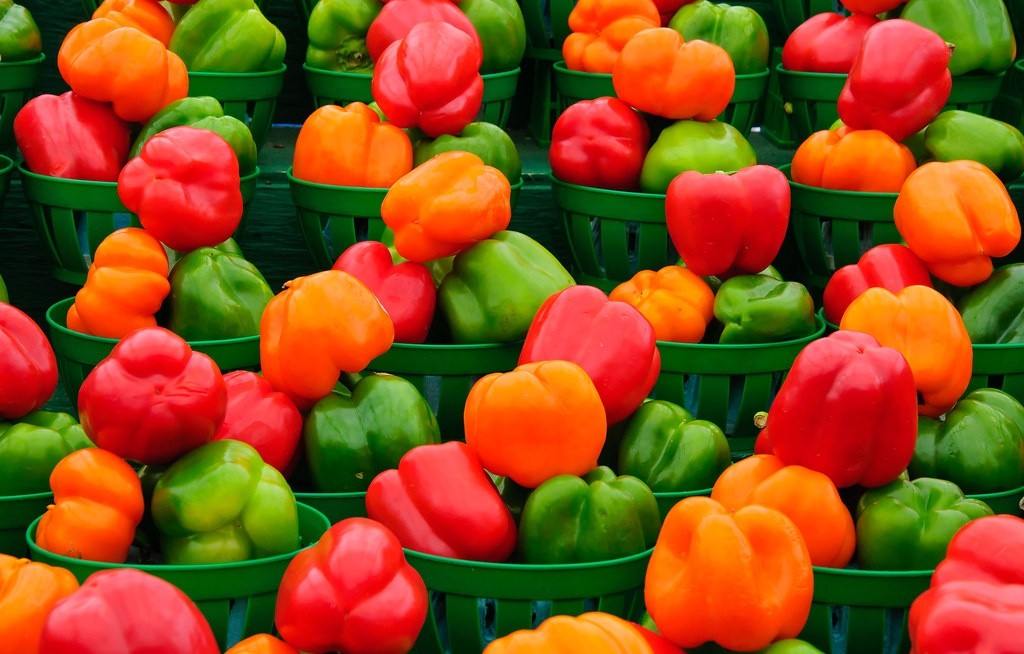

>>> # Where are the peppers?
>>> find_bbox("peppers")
[0,0,1024,654]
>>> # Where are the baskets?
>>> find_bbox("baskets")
[0,0,1024,654]
